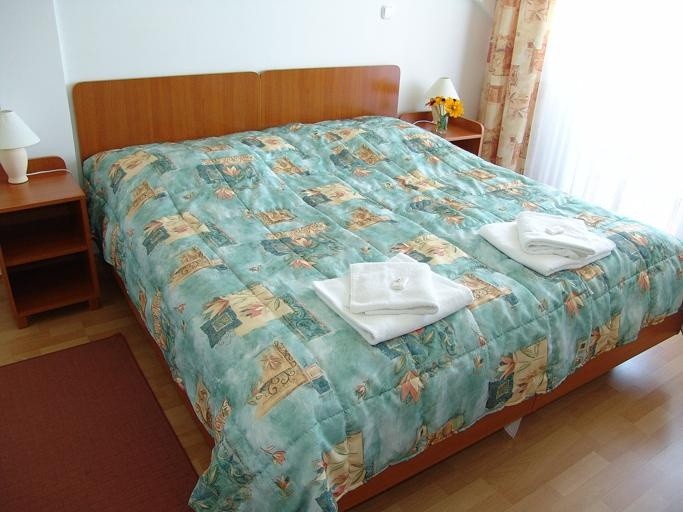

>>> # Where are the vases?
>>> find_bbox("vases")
[435,115,448,134]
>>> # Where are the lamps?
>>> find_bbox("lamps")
[425,77,460,124]
[1,108,39,184]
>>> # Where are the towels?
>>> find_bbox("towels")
[311,252,474,345]
[478,210,617,278]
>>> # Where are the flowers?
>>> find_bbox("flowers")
[425,95,465,130]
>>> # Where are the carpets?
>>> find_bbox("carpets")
[1,332,202,512]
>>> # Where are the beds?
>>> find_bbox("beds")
[71,64,683,511]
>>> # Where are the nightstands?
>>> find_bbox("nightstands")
[0,156,103,329]
[398,110,485,158]
